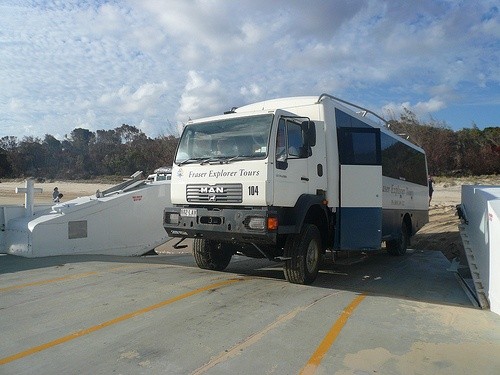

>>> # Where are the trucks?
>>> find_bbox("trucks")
[145,171,171,184]
[162,93,429,286]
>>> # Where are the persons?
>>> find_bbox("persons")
[272,130,298,157]
[52,187,62,202]
